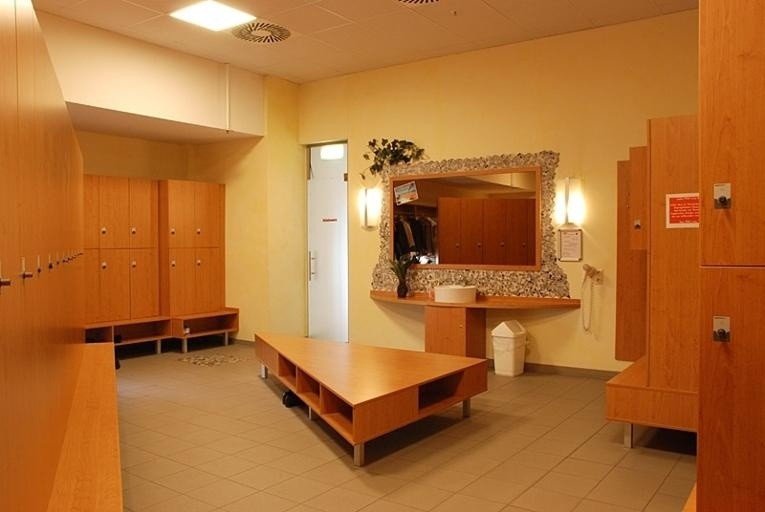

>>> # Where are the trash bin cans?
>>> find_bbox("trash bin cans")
[491,319,526,377]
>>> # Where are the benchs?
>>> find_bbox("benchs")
[252,330,490,468]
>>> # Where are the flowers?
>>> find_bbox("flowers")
[359,136,424,180]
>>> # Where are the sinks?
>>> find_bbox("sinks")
[433,285,477,302]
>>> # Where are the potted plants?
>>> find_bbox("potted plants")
[386,253,421,298]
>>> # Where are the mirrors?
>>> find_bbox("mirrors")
[368,148,571,299]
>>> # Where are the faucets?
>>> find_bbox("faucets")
[458,273,469,286]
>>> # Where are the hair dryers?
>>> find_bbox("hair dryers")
[582,264,597,278]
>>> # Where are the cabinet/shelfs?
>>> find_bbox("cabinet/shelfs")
[2,0,86,512]
[87,307,240,360]
[86,174,224,322]
[684,7,764,512]
[606,112,701,451]
[436,195,536,266]
[423,304,486,361]
